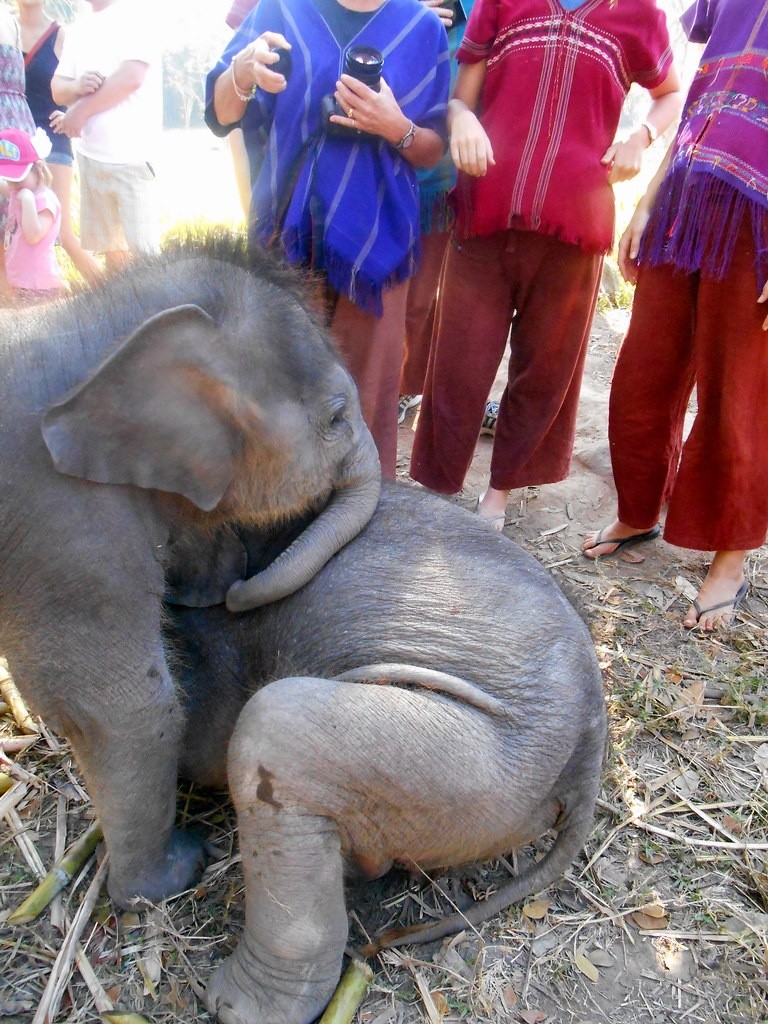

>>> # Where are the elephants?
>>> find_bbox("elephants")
[3,232,384,914]
[161,477,608,1024]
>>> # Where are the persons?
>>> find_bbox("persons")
[0,0,167,309]
[206,0,500,486]
[579,0,768,630]
[410,0,682,533]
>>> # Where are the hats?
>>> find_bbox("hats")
[0,128,40,183]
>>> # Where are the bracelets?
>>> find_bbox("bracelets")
[231,56,256,101]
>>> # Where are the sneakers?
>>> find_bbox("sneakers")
[479,401,503,434]
[397,393,422,424]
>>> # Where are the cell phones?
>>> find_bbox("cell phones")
[437,0,467,34]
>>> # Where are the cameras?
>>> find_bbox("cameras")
[321,47,385,137]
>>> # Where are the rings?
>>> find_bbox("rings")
[352,121,354,128]
[348,108,353,117]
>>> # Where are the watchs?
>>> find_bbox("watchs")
[641,121,657,147]
[393,121,415,151]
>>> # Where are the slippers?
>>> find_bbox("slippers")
[476,493,506,532]
[684,579,749,634]
[583,524,660,561]
